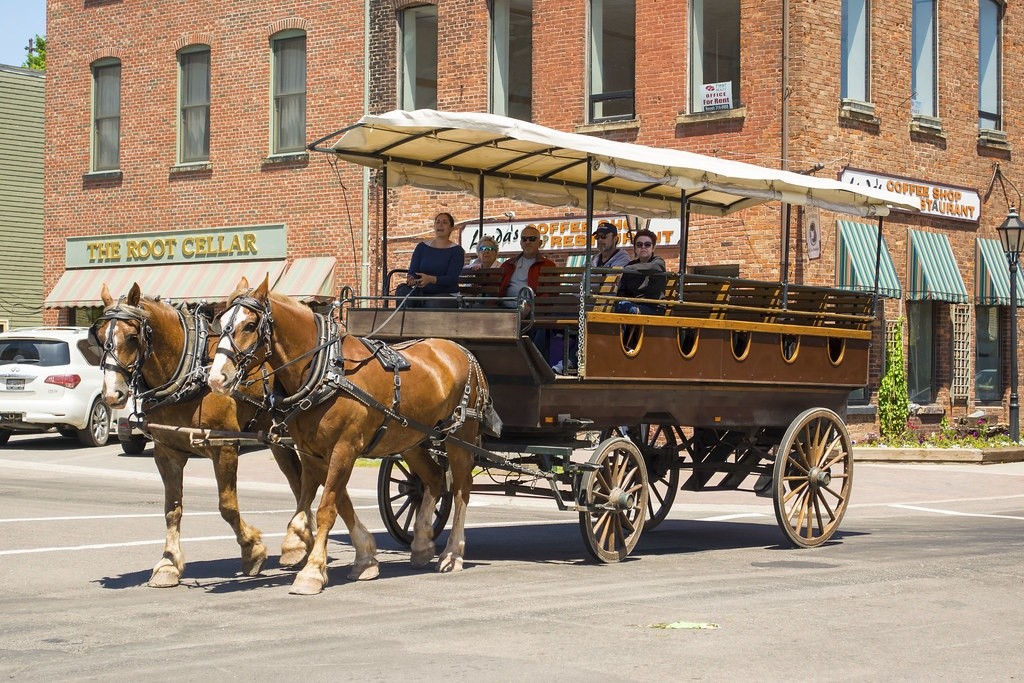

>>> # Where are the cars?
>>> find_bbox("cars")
[113,383,269,458]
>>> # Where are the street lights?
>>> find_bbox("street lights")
[994,201,1024,444]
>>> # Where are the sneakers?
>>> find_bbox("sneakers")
[551,359,574,375]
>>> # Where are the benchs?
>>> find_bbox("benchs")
[821,291,875,330]
[727,282,784,323]
[458,267,505,308]
[774,285,831,359]
[521,266,623,375]
[666,279,732,319]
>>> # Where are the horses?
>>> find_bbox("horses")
[208,272,490,595]
[91,281,317,586]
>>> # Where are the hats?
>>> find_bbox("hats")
[593,223,617,236]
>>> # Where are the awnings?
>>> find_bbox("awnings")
[907,229,968,304]
[45,257,336,307]
[975,238,1024,307]
[834,221,902,299]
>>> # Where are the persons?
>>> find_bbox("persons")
[463,236,503,305]
[535,222,666,373]
[395,213,464,308]
[472,225,559,310]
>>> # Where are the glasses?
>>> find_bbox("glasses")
[522,236,539,242]
[478,246,496,252]
[594,234,614,239]
[635,242,654,248]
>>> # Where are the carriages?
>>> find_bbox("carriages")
[84,106,925,595]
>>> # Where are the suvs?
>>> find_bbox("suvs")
[0,326,144,447]
